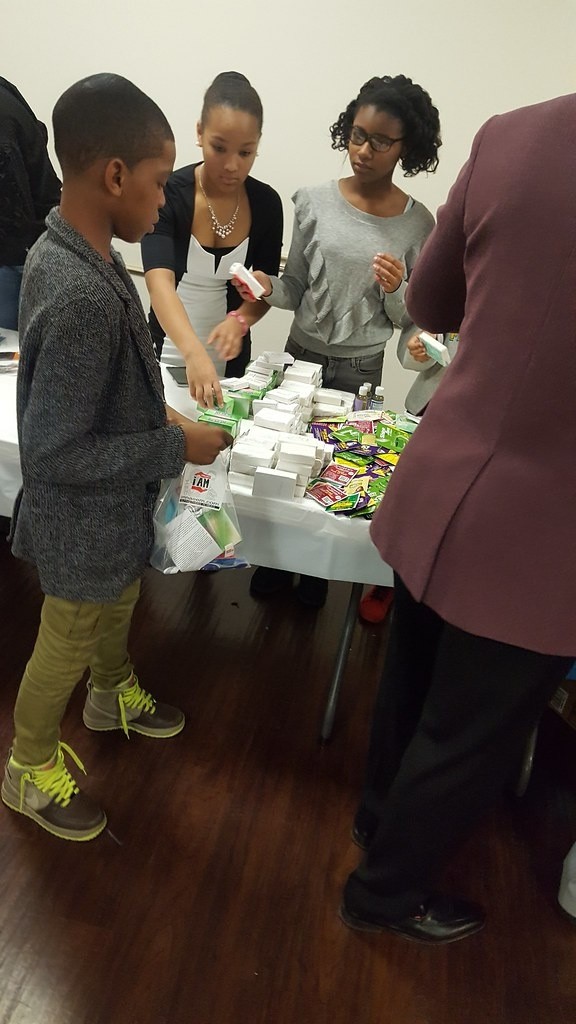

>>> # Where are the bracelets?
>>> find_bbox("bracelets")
[227,311,248,336]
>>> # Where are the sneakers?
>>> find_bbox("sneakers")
[339,815,488,947]
[83,670,186,739]
[1,742,108,843]
[359,584,396,631]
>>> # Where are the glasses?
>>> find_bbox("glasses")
[346,125,406,153]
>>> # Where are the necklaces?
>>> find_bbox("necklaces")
[199,162,240,238]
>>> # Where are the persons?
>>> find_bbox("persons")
[361,320,461,622]
[232,74,443,617]
[141,71,283,408]
[337,93,576,941]
[1,72,232,842]
[0,76,62,330]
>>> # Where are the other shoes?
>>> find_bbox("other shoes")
[249,566,329,616]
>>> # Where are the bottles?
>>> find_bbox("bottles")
[354,382,384,412]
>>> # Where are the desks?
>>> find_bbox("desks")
[0,324,426,742]
